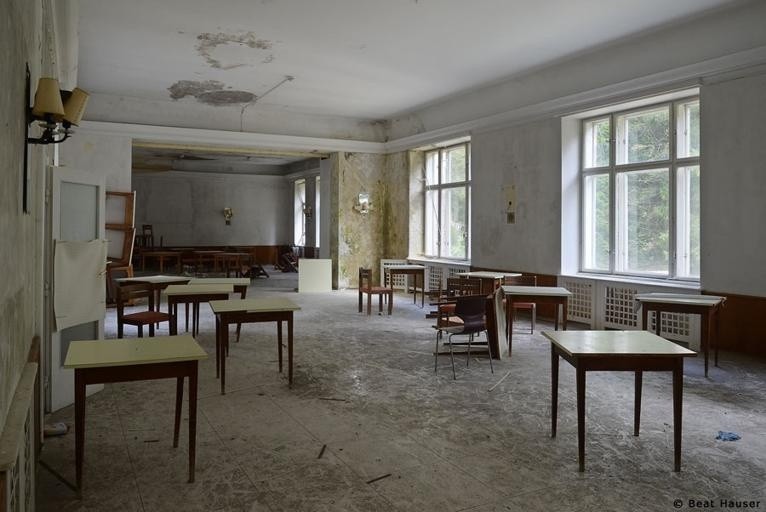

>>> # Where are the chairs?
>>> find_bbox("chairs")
[358,269,393,317]
[132,223,159,268]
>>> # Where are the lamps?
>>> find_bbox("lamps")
[23,61,90,213]
[352,188,374,215]
